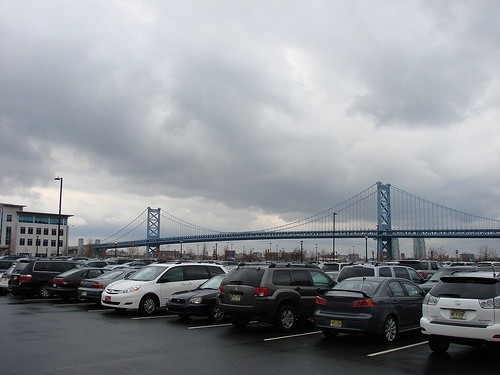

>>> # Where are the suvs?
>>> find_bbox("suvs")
[101,260,229,320]
[419,270,500,353]
[217,261,338,334]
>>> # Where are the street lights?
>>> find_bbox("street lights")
[300,240,303,264]
[227,242,229,251]
[352,246,355,255]
[230,244,233,250]
[53,176,63,256]
[332,212,338,261]
[276,245,278,252]
[270,243,272,253]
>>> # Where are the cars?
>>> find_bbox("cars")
[314,276,427,347]
[0,255,500,310]
[166,274,227,324]
